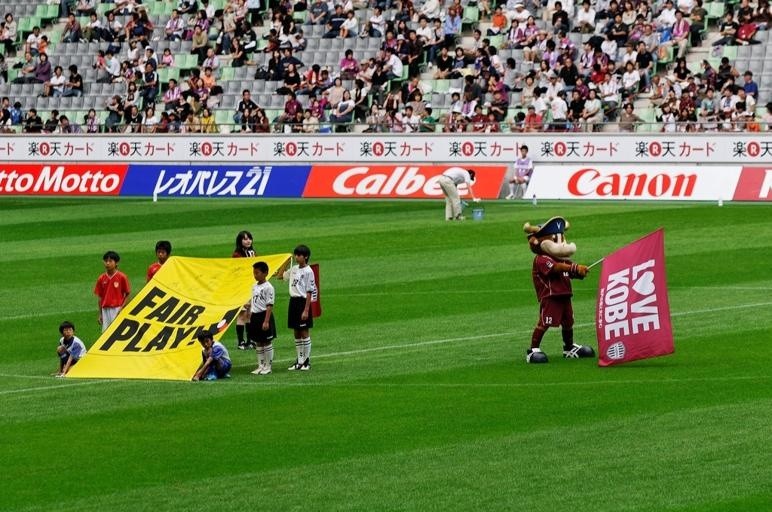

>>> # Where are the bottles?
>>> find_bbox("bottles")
[718,196,722,206]
[532,194,536,205]
[462,200,470,207]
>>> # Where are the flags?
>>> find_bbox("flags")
[595,227,675,367]
[63,252,293,381]
[309,264,322,318]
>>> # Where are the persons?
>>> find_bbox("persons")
[93,250,132,334]
[248,261,277,374]
[145,240,172,284]
[0,1,772,134]
[503,144,534,199]
[437,166,481,221]
[276,244,315,371]
[232,229,258,348]
[49,321,88,378]
[191,330,233,382]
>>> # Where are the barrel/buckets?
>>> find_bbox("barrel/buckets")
[471,201,486,222]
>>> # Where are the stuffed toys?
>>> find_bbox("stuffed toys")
[523,216,595,363]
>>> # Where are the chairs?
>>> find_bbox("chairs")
[0,0,772,136]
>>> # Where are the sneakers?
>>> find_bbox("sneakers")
[288,358,310,371]
[239,339,256,350]
[252,366,271,374]
